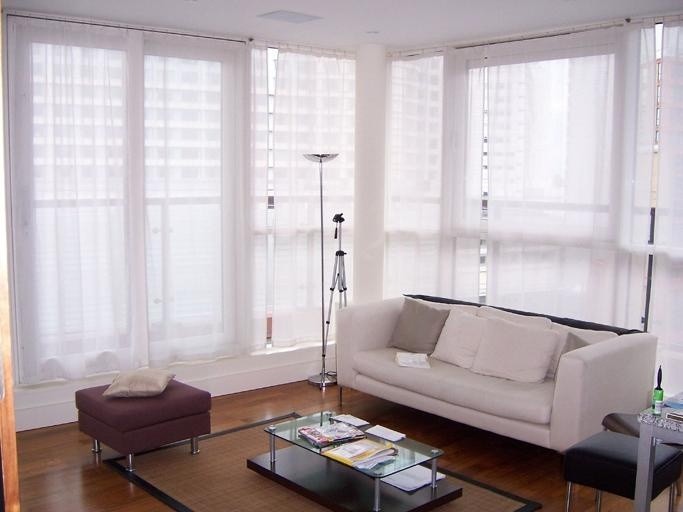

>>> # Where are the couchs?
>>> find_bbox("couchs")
[333,294,655,457]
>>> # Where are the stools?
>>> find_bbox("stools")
[74,379,213,472]
[595,413,683,512]
[554,428,682,512]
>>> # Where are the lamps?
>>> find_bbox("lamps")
[298,152,344,387]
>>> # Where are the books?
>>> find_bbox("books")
[393,351,430,370]
[666,408,683,422]
[297,419,398,469]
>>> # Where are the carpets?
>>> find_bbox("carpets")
[100,413,542,512]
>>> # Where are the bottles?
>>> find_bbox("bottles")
[650,365,664,417]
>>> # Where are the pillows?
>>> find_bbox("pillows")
[389,297,592,385]
[100,365,179,400]
